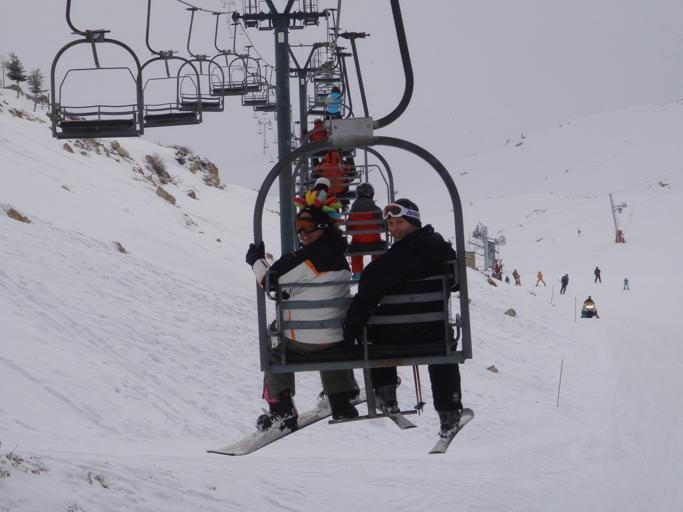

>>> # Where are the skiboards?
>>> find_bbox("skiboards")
[365,387,475,453]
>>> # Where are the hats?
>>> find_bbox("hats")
[393,198,421,228]
[356,182,374,197]
[315,177,330,187]
[297,206,331,229]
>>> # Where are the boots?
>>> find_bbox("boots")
[328,394,359,420]
[375,384,398,413]
[255,399,299,431]
[436,410,463,433]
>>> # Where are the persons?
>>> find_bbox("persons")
[495,264,629,318]
[343,198,462,437]
[290,86,349,224]
[246,205,359,430]
[346,183,388,281]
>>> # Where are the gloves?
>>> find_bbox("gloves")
[343,323,362,344]
[245,241,265,266]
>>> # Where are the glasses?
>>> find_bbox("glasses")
[295,218,320,234]
[383,204,406,220]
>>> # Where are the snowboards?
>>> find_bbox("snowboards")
[207,376,400,455]
